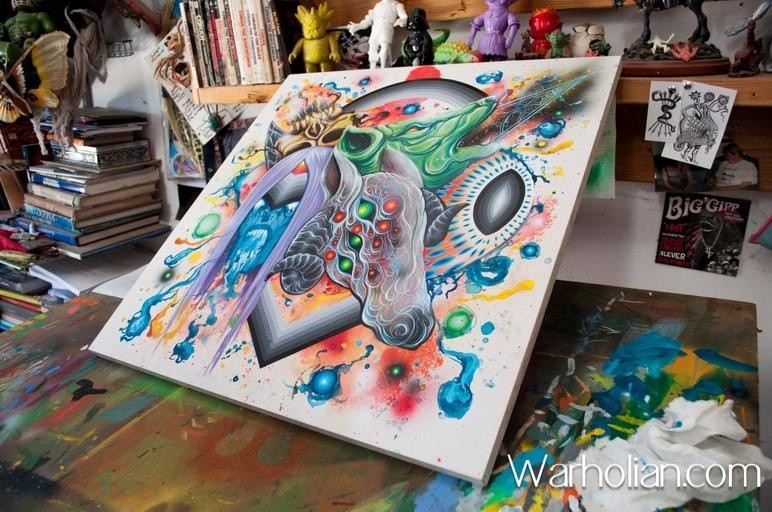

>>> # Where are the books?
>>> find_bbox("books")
[178,0,314,88]
[0,107,171,332]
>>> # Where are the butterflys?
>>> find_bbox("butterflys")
[0,30,71,123]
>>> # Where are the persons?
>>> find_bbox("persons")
[661,143,760,193]
